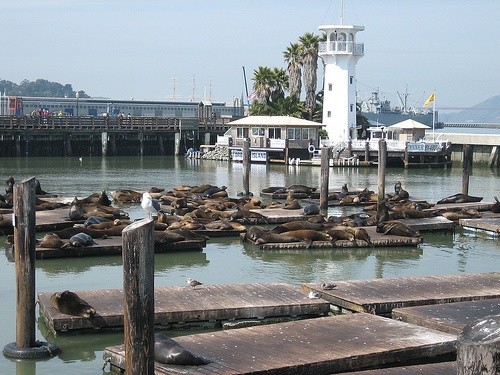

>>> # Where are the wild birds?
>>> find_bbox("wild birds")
[140,193,160,220]
[186,278,203,288]
[308,290,322,299]
[322,281,338,290]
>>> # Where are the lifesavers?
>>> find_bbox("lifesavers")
[307,144,316,153]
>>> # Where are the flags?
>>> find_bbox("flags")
[425,92,435,107]
